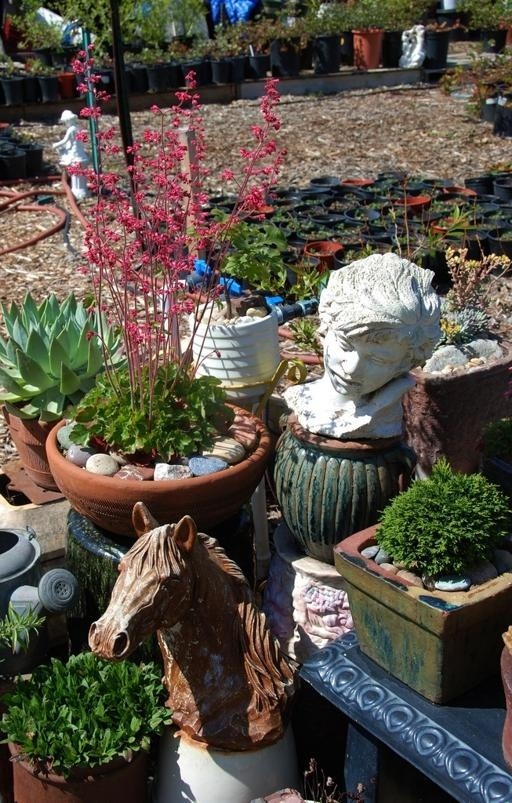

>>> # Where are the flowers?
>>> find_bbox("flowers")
[66,38,287,458]
[414,242,512,375]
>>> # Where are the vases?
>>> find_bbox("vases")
[46,403,270,535]
[274,417,414,563]
[500,625,512,773]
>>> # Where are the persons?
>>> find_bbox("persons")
[279,252,441,440]
[52,109,90,189]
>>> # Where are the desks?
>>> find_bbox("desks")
[298,630,512,803]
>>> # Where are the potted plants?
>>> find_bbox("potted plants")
[0,293,128,491]
[333,459,512,702]
[0,124,44,179]
[0,650,175,803]
[186,206,282,403]
[279,315,325,364]
